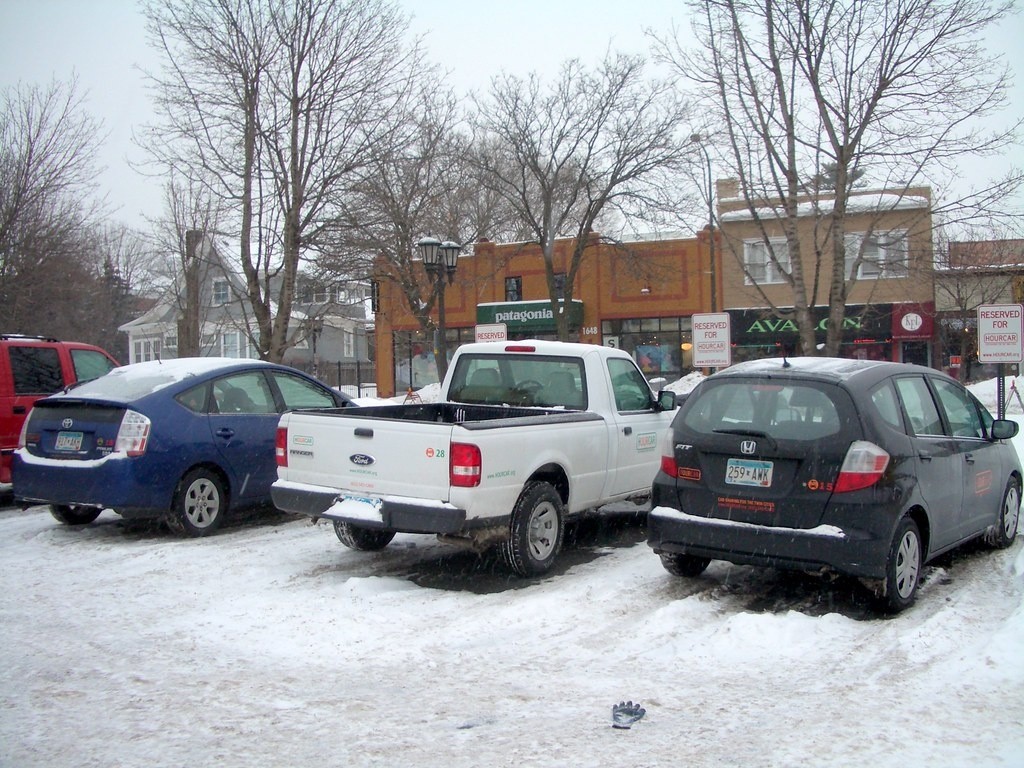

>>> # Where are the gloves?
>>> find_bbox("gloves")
[612,701,646,731]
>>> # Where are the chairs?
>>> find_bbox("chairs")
[546,371,578,405]
[468,369,501,401]
[778,386,826,439]
[223,388,253,413]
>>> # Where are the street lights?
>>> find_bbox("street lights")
[416,236,462,382]
[690,132,716,312]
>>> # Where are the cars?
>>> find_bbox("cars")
[646,356,1024,613]
[11,357,362,538]
[270,321,681,578]
[2,336,119,500]
